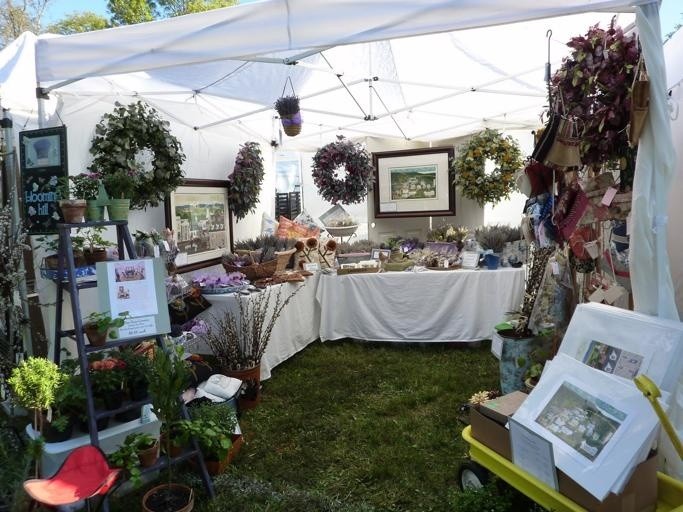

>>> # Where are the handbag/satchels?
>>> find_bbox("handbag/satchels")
[627,80,648,147]
[517,113,632,306]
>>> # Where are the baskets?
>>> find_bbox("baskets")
[223,247,297,279]
[280,113,301,136]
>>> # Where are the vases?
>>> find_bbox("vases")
[498,329,545,395]
[525,377,534,394]
[223,365,259,408]
[326,226,357,237]
[487,255,498,270]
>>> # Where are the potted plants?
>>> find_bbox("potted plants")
[126,334,194,512]
[86,169,131,224]
[192,400,242,474]
[389,240,400,255]
[41,176,87,224]
[6,224,241,432]
[112,427,160,485]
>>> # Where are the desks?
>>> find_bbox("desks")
[191,270,321,382]
[316,264,527,343]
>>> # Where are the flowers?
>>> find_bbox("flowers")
[87,98,186,211]
[310,134,378,208]
[273,97,307,137]
[227,140,265,219]
[448,128,525,209]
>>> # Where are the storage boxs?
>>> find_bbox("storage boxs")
[470,390,660,512]
[491,320,521,361]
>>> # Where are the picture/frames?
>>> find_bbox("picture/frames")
[370,145,458,218]
[371,249,391,260]
[165,179,233,274]
